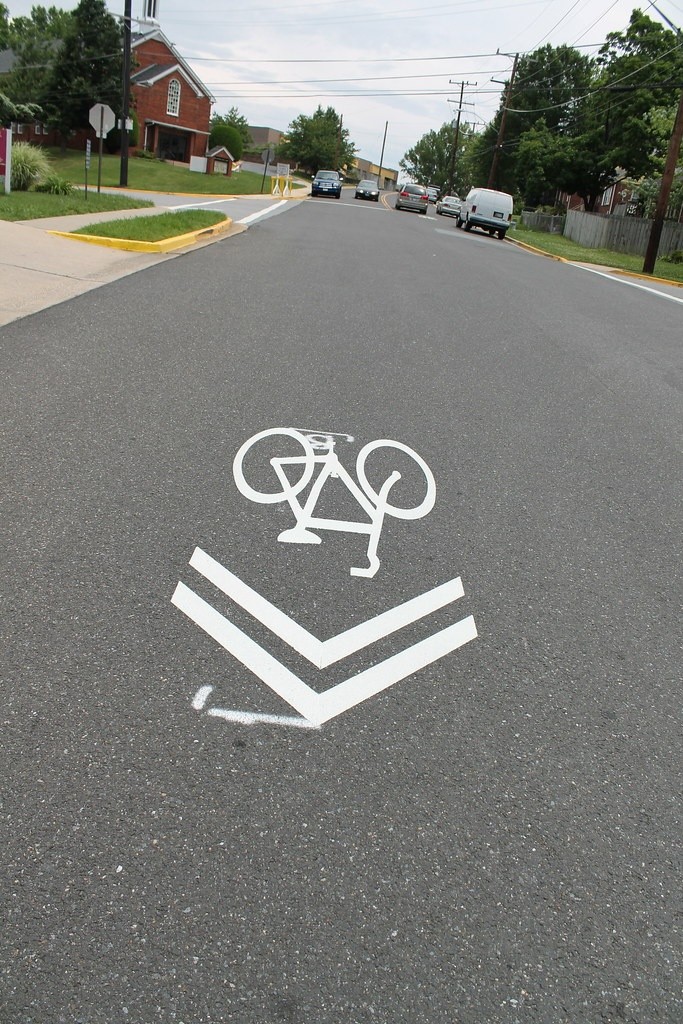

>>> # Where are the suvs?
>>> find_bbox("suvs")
[394,184,431,214]
[311,169,343,199]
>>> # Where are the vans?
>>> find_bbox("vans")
[454,187,515,240]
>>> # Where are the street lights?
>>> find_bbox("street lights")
[451,108,501,190]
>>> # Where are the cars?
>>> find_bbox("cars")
[426,188,438,205]
[353,179,381,202]
[435,196,463,217]
